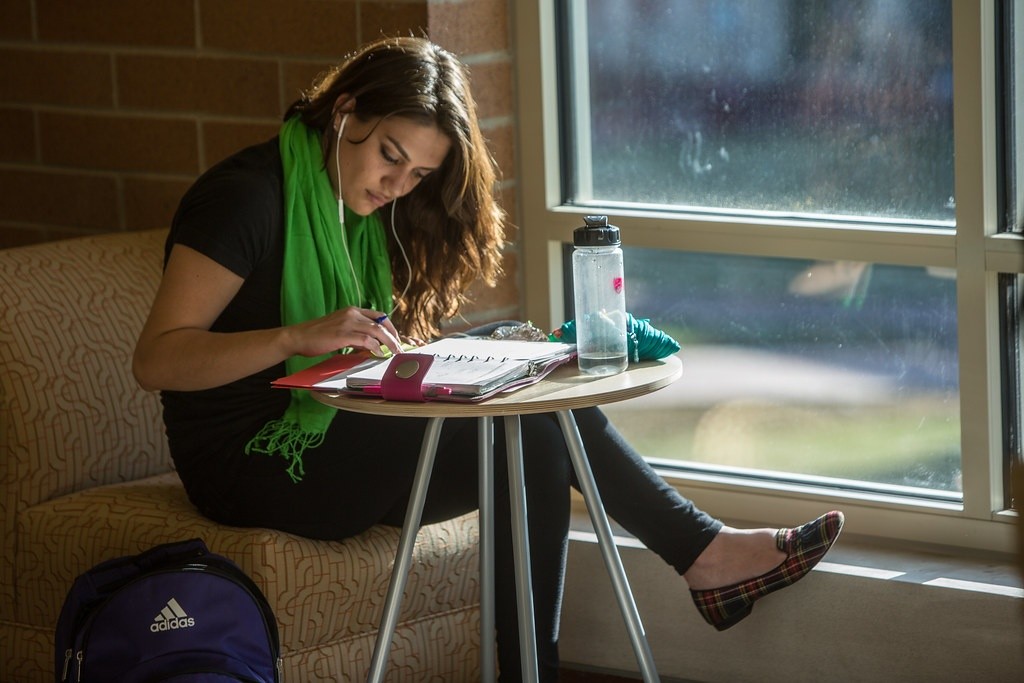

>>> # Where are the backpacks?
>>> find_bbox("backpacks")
[55,538,282,683]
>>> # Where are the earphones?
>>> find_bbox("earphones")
[337,112,349,139]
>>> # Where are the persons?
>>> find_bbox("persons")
[129,36,845,683]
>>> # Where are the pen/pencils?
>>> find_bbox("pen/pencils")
[375,315,390,327]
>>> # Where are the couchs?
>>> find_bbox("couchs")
[0,227,501,683]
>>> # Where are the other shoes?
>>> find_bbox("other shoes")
[687,510,844,633]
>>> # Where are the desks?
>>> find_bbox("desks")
[314,355,684,683]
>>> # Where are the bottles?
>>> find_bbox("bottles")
[572,215,628,377]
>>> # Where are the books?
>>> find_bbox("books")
[271,336,577,402]
